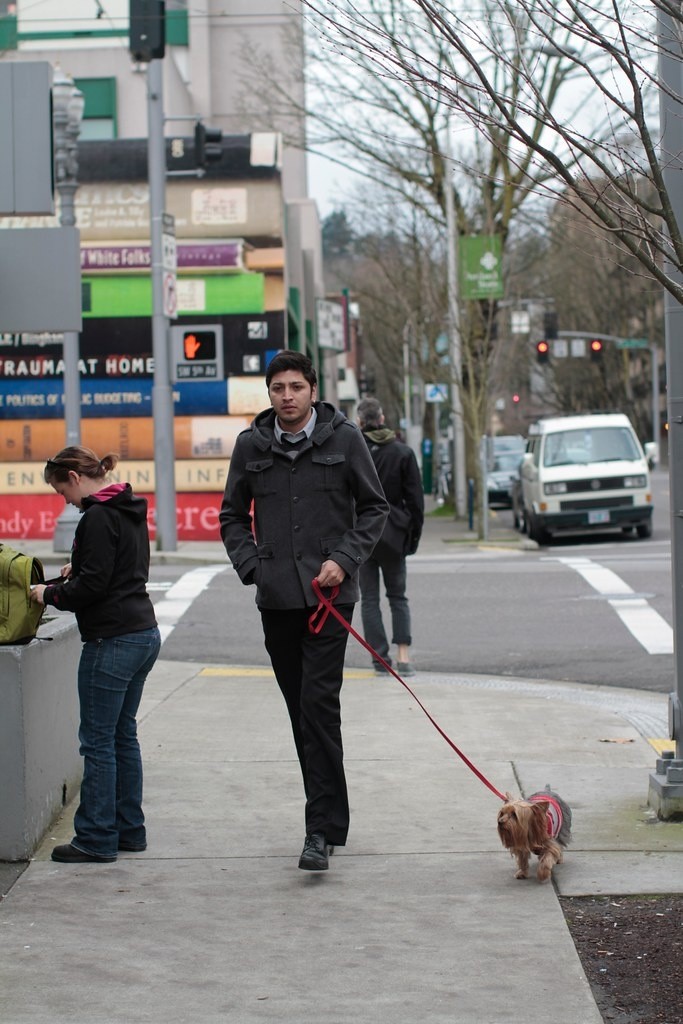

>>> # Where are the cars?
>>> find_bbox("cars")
[486,450,521,504]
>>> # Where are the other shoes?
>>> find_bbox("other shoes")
[117,826,148,852]
[374,665,391,675]
[397,662,416,676]
[50,843,117,863]
[298,833,333,870]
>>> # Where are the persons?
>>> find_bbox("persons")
[28,446,162,861]
[356,397,426,676]
[219,350,390,869]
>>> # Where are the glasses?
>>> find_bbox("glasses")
[45,458,85,476]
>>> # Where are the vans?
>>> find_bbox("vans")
[518,415,659,543]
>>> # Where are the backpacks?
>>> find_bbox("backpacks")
[0,544,68,644]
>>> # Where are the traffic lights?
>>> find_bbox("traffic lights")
[512,395,521,408]
[592,338,604,361]
[536,338,549,361]
[195,124,225,170]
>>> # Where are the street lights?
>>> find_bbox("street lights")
[52,69,83,553]
[445,44,577,519]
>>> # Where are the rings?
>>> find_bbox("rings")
[328,583,331,586]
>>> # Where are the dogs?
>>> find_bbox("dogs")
[496,783,573,884]
